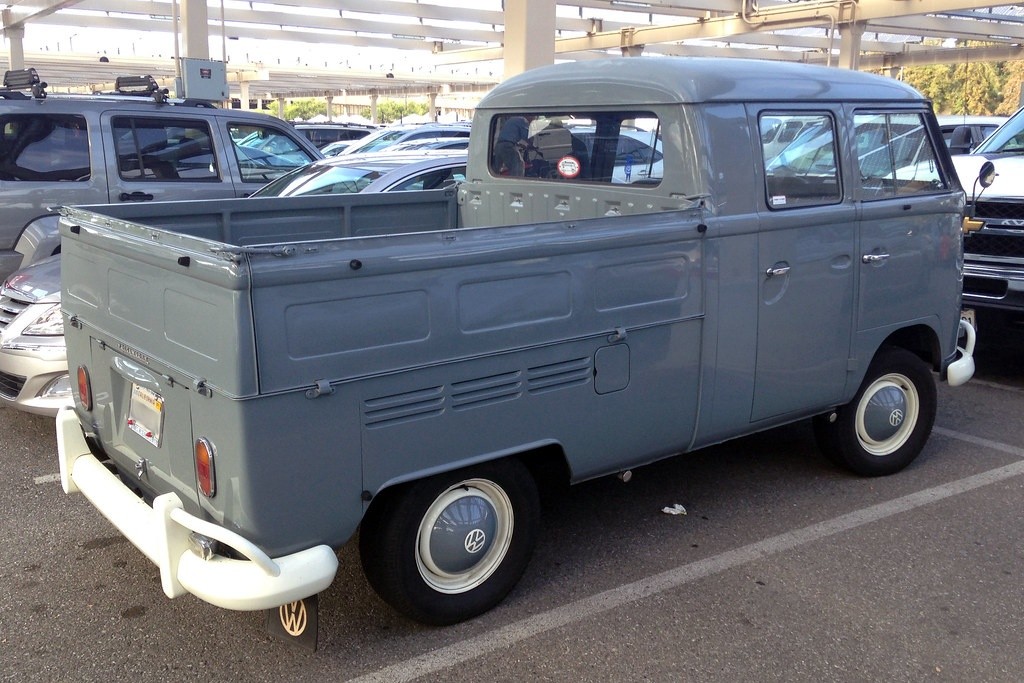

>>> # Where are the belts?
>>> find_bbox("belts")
[497,140,515,145]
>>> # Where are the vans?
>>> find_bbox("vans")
[758,114,1023,216]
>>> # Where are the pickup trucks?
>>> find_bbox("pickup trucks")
[57,54,1001,658]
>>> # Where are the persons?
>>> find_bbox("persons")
[494,116,535,177]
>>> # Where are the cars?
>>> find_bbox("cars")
[237,103,678,201]
[1,152,474,426]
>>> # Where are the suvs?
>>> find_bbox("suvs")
[0,69,328,288]
[887,106,1024,330]
